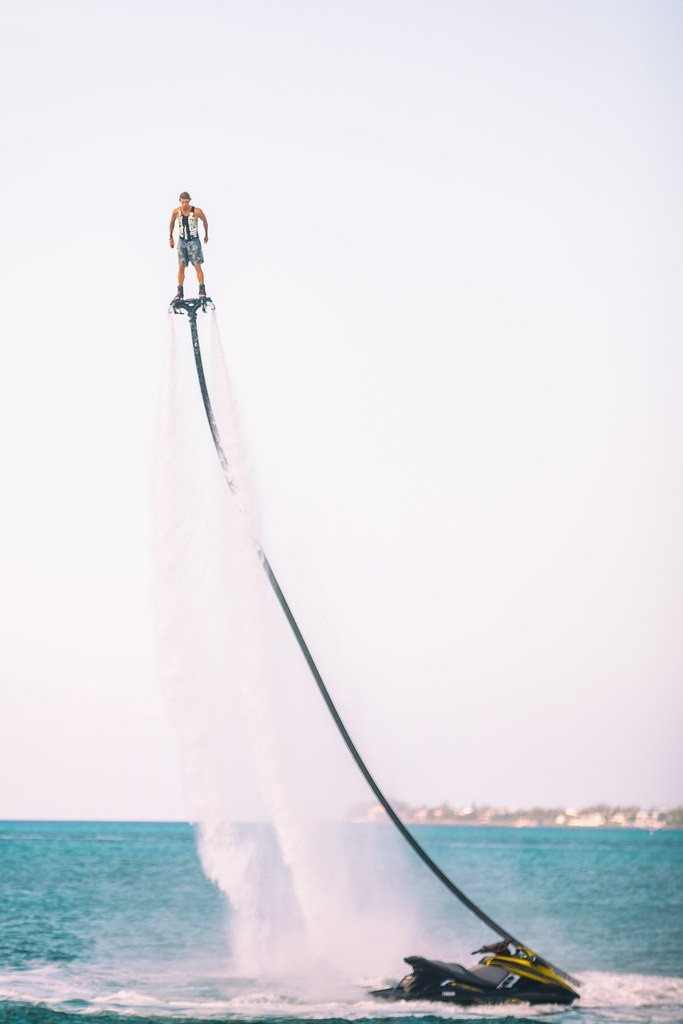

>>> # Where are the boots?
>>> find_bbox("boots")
[199,285,205,297]
[176,286,183,299]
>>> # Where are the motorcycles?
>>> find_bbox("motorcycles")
[368,937,581,1007]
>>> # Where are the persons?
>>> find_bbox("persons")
[168,191,208,299]
